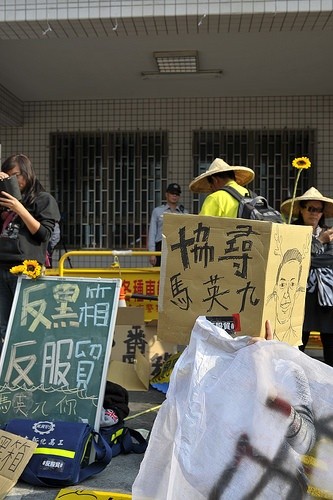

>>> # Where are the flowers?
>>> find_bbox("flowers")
[9,259,43,281]
[287,156,312,225]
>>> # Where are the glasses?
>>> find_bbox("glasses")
[302,206,325,214]
[167,191,180,196]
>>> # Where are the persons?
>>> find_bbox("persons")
[188,157,259,221]
[147,184,190,267]
[280,186,333,368]
[0,152,60,363]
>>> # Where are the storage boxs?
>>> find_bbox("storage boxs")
[155,212,313,351]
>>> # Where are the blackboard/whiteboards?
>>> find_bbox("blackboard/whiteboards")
[0,271,122,471]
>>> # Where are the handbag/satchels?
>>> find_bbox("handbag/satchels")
[102,418,146,458]
[0,418,114,490]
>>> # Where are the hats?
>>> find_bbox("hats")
[281,187,333,220]
[166,183,182,193]
[188,158,256,194]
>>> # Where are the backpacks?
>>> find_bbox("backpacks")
[218,185,284,223]
